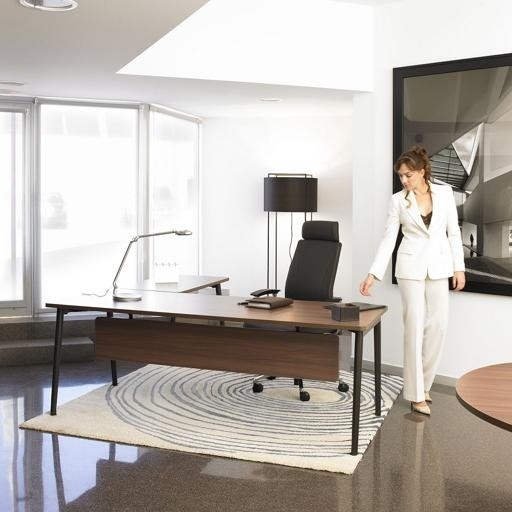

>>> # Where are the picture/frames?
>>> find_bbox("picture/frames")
[391,53,512,297]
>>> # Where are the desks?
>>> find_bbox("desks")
[452,363,512,431]
[45,273,388,456]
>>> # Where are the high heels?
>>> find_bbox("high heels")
[410,392,432,414]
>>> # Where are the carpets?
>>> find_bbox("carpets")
[18,363,405,476]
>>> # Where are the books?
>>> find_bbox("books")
[245,296,293,310]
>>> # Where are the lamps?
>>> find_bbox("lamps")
[262,172,317,297]
[109,229,193,302]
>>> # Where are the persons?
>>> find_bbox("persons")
[400,405,446,512]
[360,146,465,415]
[470,233,474,247]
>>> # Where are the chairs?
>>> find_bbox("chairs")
[247,218,342,400]
